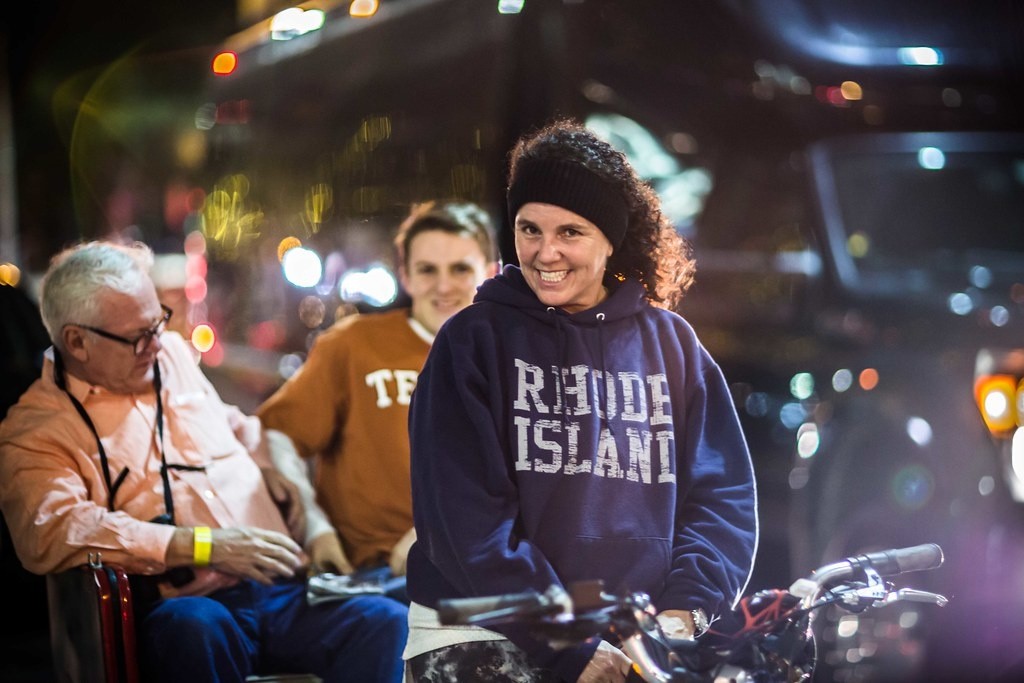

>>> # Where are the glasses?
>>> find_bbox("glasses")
[62,297,174,351]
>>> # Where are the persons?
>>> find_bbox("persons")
[403,114,760,683]
[255,200,504,580]
[0,237,413,683]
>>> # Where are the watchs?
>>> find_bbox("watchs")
[691,606,709,639]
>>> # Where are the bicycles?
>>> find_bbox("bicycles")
[428,546,946,682]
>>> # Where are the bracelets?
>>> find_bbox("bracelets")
[193,525,212,569]
